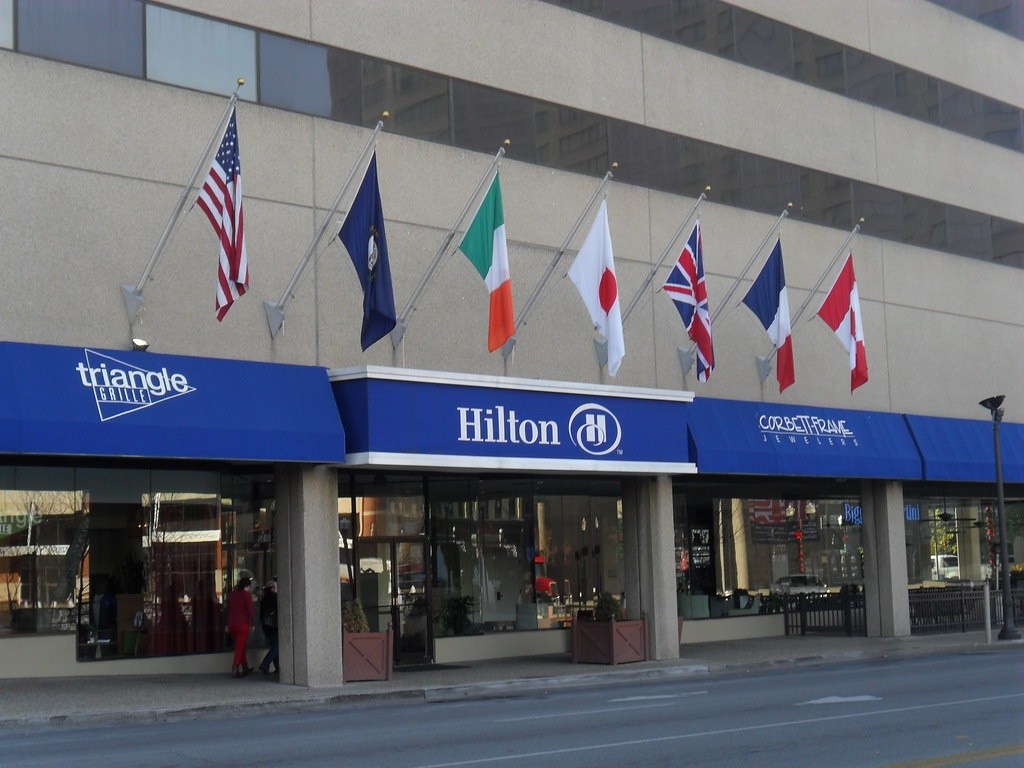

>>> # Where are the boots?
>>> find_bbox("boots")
[232,665,243,678]
[242,664,253,675]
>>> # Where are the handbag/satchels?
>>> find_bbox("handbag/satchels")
[264,609,278,628]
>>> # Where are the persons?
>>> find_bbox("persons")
[258,582,279,675]
[226,578,257,677]
[160,583,221,655]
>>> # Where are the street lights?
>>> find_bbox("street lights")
[338,517,355,598]
[978,393,1022,641]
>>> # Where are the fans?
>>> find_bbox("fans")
[967,521,997,529]
[916,494,977,522]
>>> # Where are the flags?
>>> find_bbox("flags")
[197,106,249,322]
[662,219,716,384]
[818,253,869,394]
[339,152,396,352]
[741,236,795,395]
[458,169,515,353]
[567,198,625,377]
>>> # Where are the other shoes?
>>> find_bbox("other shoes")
[258,665,270,675]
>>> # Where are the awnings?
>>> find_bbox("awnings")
[903,414,1024,483]
[687,398,922,481]
[0,341,347,463]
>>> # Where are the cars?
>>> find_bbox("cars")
[773,574,829,598]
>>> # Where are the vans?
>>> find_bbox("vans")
[931,554,959,581]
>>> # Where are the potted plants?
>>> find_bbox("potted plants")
[570,591,648,666]
[342,599,393,683]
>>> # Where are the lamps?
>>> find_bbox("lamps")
[132,337,148,352]
